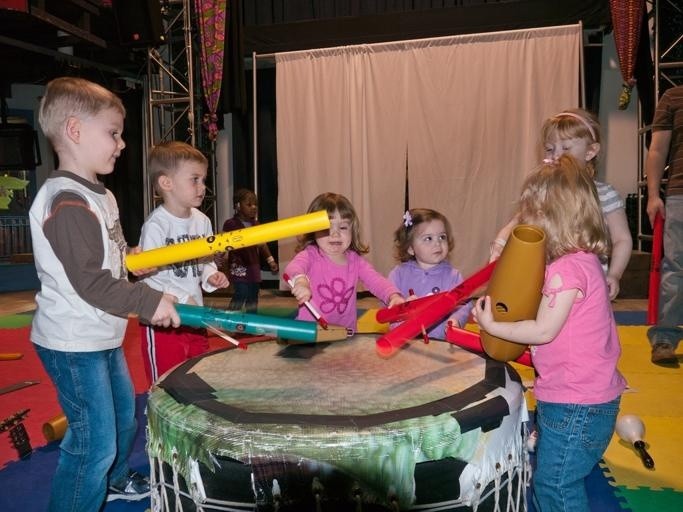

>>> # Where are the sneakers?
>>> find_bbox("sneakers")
[106,468,151,504]
[650,342,678,363]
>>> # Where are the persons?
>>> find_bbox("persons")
[284,192,406,333]
[645,85,683,364]
[490,107,633,301]
[223,188,279,315]
[138,141,229,386]
[472,153,628,512]
[392,210,471,341]
[29,77,182,512]
